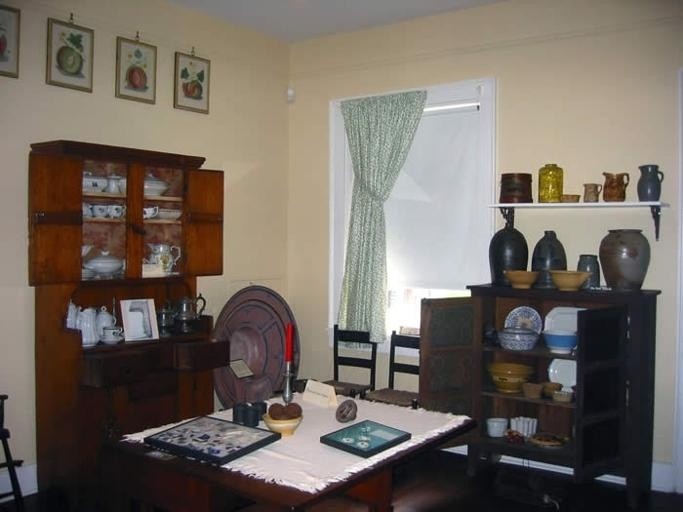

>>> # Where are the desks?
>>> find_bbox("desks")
[112,388,477,512]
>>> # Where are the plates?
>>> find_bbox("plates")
[503,306,543,337]
[101,337,123,345]
[81,270,94,280]
[543,307,586,350]
[548,360,576,388]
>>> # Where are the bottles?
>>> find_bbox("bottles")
[488,223,528,285]
[531,229,566,290]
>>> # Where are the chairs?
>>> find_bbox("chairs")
[318,324,376,403]
[359,332,420,407]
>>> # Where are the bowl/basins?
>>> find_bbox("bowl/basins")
[487,363,536,394]
[82,177,108,193]
[156,208,181,219]
[262,413,302,438]
[544,269,594,292]
[496,328,539,353]
[83,250,123,275]
[502,271,539,289]
[542,330,579,355]
[119,174,169,195]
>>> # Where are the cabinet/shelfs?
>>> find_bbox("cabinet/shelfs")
[27,140,222,287]
[34,334,214,512]
[418,283,660,510]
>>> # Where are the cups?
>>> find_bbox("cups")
[107,174,122,193]
[83,203,158,219]
[233,402,267,425]
[102,326,123,338]
[582,183,602,202]
[486,417,508,437]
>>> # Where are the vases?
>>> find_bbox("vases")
[530,230,566,271]
[487,207,528,286]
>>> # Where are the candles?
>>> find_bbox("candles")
[283,323,293,362]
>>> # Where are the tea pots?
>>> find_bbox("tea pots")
[602,172,629,203]
[165,292,206,323]
[66,298,99,347]
[147,241,181,273]
[96,305,116,337]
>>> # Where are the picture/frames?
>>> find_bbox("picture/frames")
[114,35,157,105]
[318,418,410,457]
[45,16,95,93]
[0,4,22,79]
[173,50,211,115]
[141,414,283,466]
